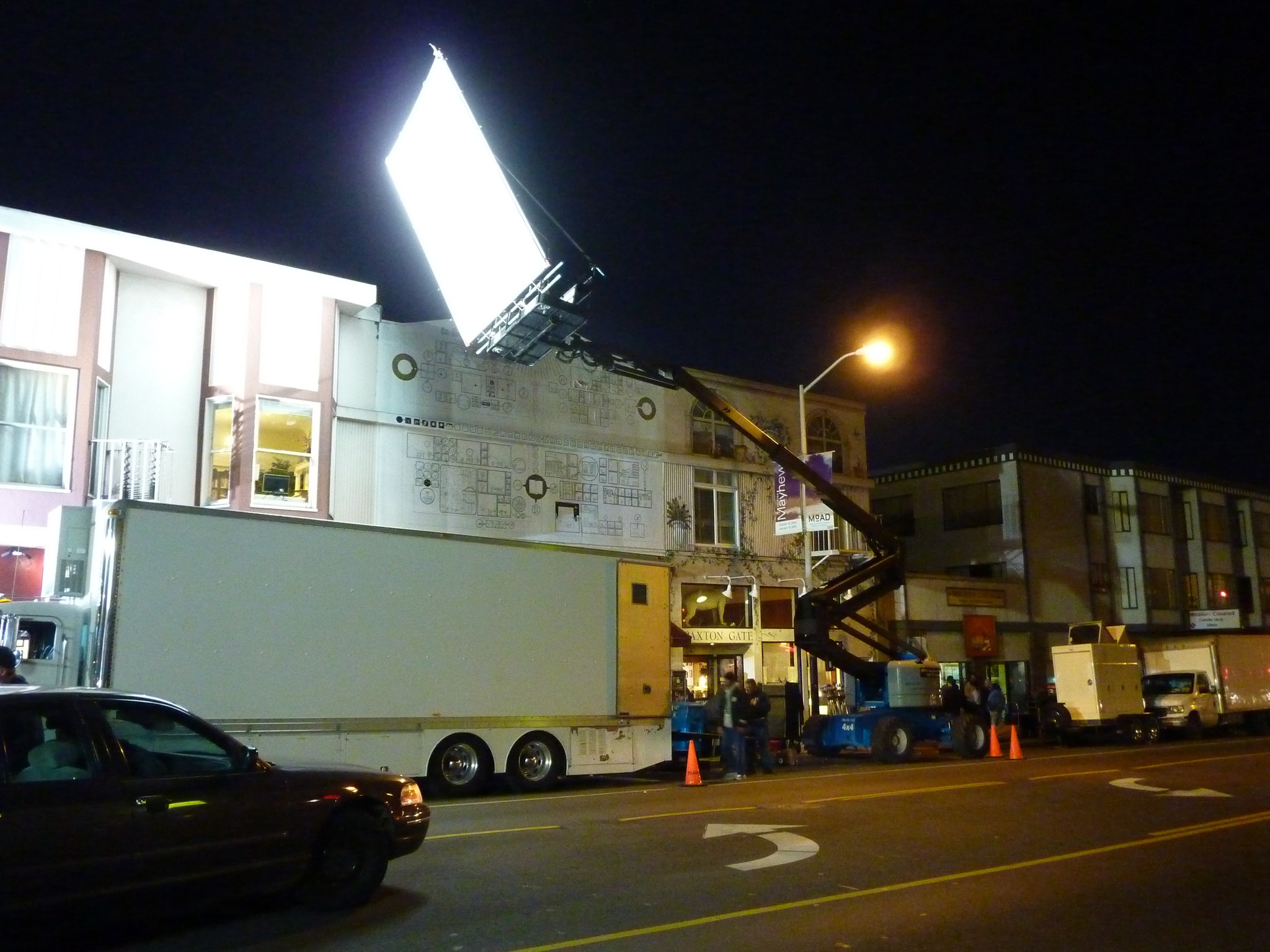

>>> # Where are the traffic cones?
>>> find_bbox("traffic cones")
[681,740,708,788]
[1004,724,1027,760]
[984,724,1006,759]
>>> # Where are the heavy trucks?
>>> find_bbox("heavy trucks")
[2,498,673,797]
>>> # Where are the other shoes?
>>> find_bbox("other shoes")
[733,774,746,780]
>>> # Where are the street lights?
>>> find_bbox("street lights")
[799,335,901,726]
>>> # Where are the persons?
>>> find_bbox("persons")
[0,645,46,765]
[941,674,1006,726]
[713,671,745,780]
[731,678,774,780]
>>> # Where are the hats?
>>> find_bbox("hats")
[0,646,16,668]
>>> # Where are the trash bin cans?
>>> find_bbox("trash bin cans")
[673,702,703,756]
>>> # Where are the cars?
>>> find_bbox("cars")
[0,683,429,923]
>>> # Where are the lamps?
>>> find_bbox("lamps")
[704,575,733,599]
[778,578,806,598]
[728,575,758,598]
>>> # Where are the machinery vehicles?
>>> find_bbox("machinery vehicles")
[380,41,993,764]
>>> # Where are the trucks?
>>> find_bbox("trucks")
[1141,634,1270,738]
